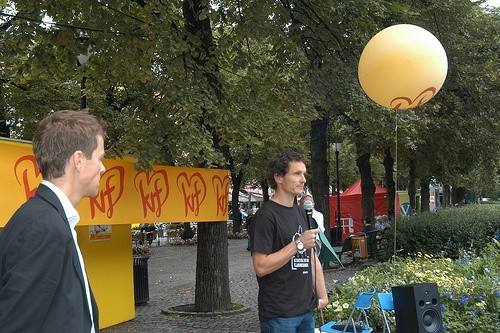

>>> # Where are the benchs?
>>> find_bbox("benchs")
[364,226,390,260]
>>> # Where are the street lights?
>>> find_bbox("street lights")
[329,134,344,246]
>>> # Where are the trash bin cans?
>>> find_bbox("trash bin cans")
[133,254,151,305]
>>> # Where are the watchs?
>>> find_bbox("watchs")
[295,239,304,252]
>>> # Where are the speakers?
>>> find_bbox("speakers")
[392,282,444,333]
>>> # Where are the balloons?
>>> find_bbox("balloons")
[358,23,447,110]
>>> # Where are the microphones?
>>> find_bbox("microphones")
[303,200,313,230]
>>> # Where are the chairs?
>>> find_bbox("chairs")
[377,293,396,333]
[335,235,355,262]
[319,286,376,333]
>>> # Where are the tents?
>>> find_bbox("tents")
[329,178,400,243]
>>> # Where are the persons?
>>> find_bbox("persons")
[247,210,254,250]
[0,111,106,333]
[250,152,328,333]
[300,195,324,269]
[376,215,390,228]
